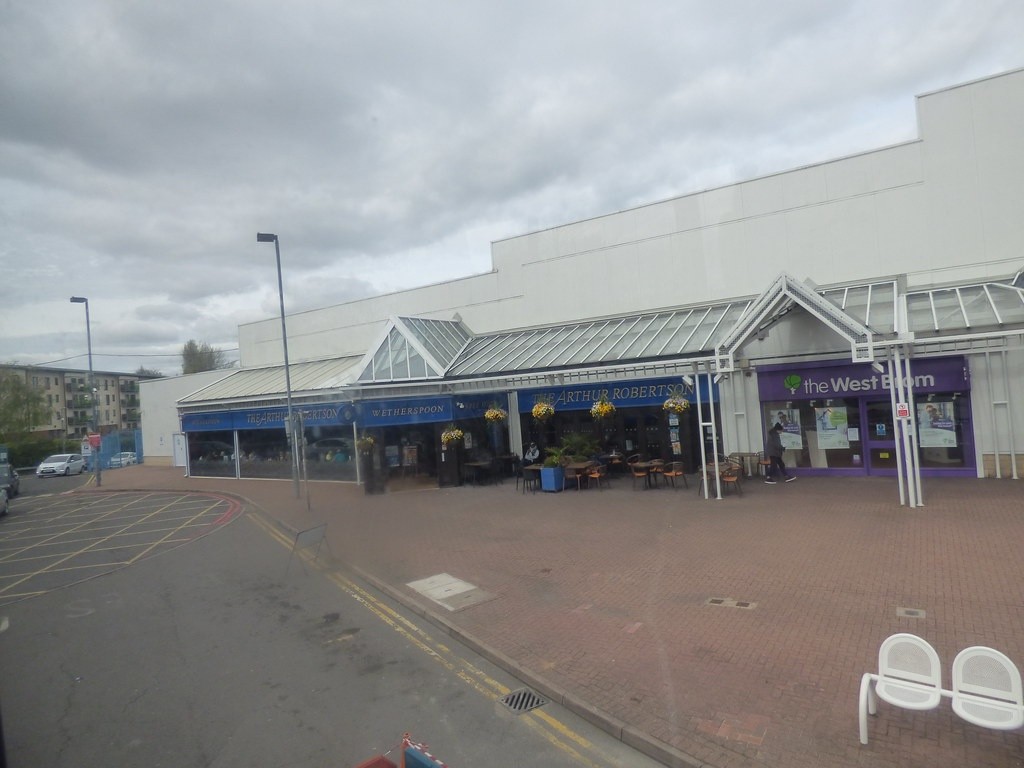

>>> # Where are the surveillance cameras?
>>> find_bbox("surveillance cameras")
[459,405,464,409]
[724,375,729,380]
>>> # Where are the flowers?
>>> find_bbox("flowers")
[441,425,466,449]
[663,396,691,415]
[532,401,559,425]
[589,398,616,427]
[357,432,375,452]
[485,406,507,424]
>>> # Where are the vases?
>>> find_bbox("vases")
[449,439,460,448]
[599,414,613,427]
[538,415,552,424]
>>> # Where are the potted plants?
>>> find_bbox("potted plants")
[540,444,570,494]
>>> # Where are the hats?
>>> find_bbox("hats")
[774,422,783,430]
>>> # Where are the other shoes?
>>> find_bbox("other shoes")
[784,476,796,482]
[765,479,776,484]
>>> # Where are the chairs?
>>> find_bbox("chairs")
[463,447,772,496]
[858,633,1024,745]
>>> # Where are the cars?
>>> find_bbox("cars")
[303,437,354,463]
[0,451,20,515]
[248,442,288,462]
[107,451,138,468]
[36,453,86,479]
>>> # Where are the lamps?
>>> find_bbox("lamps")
[679,374,696,386]
[712,372,729,386]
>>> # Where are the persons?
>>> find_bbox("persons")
[400,433,409,445]
[773,411,787,431]
[198,450,346,464]
[764,422,796,484]
[920,404,941,428]
[525,441,539,463]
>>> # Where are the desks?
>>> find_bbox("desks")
[637,461,665,490]
[464,460,492,484]
[567,460,593,489]
[524,463,544,491]
[599,453,623,479]
[728,452,759,482]
[496,453,516,477]
[697,462,733,494]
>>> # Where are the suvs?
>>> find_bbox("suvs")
[189,441,245,462]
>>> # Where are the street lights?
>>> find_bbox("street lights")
[256,230,301,503]
[69,296,102,488]
